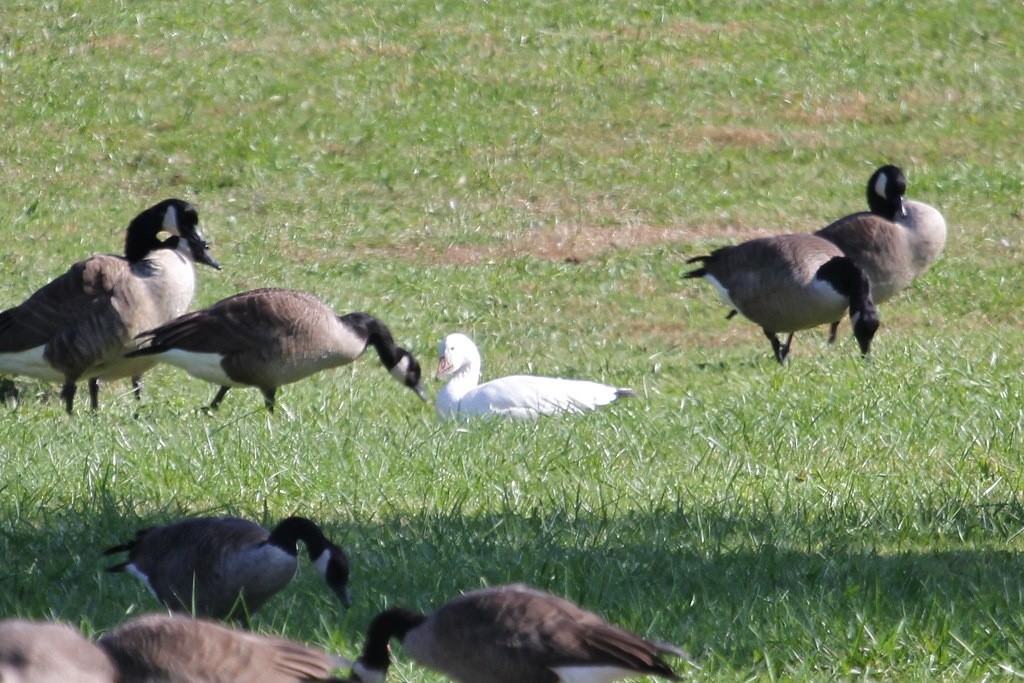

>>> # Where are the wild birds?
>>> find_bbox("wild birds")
[41,233,221,415]
[814,165,947,343]
[123,287,428,414]
[102,516,351,633]
[351,584,688,683]
[0,617,121,683]
[97,616,352,683]
[680,233,880,366]
[0,199,210,381]
[434,333,637,423]
